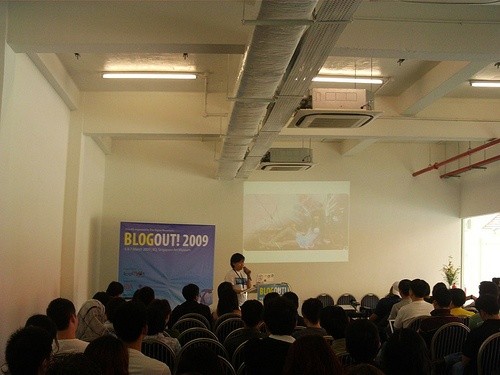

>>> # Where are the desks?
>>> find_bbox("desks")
[337,305,355,323]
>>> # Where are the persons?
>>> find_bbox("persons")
[113,301,172,375]
[394,279,435,334]
[4,280,385,375]
[47,298,90,355]
[372,277,500,374]
[225,253,252,306]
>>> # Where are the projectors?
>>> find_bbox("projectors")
[266,147,313,162]
[300,88,367,109]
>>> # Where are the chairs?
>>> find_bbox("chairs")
[338,293,356,304]
[431,322,500,375]
[317,294,334,308]
[140,313,264,375]
[360,293,379,307]
